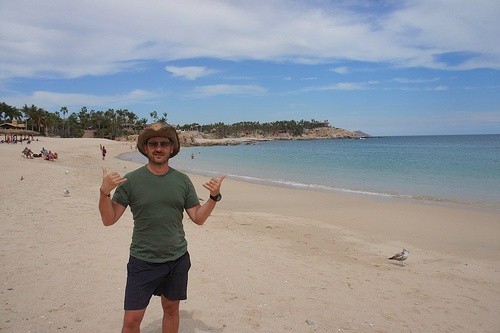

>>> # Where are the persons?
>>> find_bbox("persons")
[101,146,106,160]
[22,147,58,161]
[99,123,225,333]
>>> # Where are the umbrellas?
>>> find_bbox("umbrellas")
[0,128,41,143]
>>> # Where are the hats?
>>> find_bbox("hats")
[137,122,180,157]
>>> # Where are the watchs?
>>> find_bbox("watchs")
[210,192,222,202]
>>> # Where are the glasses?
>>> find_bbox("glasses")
[146,140,172,146]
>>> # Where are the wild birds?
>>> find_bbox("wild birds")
[389,248,410,267]
[63,187,69,197]
[20,176,24,181]
